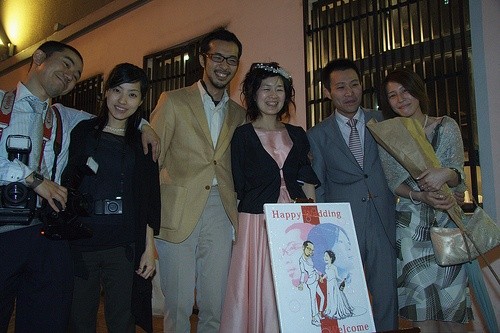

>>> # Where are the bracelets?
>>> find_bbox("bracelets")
[409,190,421,205]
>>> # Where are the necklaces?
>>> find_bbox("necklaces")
[423,114,428,129]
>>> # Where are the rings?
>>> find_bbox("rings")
[421,186,423,189]
[427,188,430,192]
[138,268,141,269]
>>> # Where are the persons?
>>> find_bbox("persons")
[63,63,161,333]
[218,63,322,333]
[378,67,500,333]
[0,39,161,333]
[308,58,465,333]
[149,29,251,333]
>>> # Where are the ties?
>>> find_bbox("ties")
[346,118,367,170]
[28,98,51,180]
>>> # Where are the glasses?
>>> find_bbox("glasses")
[204,52,239,65]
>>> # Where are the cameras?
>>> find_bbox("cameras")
[39,154,100,241]
[0,134,39,226]
[94,199,123,215]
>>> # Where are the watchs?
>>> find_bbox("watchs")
[28,173,44,191]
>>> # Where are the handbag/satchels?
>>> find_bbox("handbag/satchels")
[425,166,500,267]
